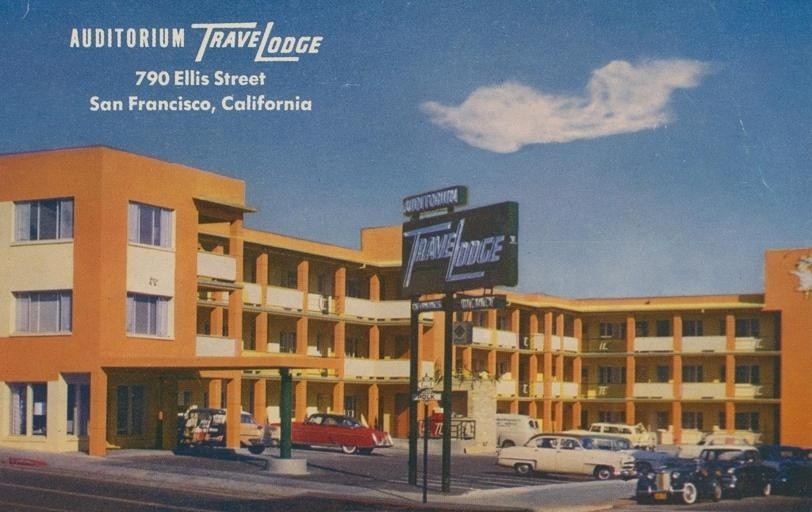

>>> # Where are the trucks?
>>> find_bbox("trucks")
[496,413,540,447]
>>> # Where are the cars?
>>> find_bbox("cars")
[177,407,272,456]
[498,416,659,479]
[268,412,394,456]
[636,431,811,505]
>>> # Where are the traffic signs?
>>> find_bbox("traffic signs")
[412,393,444,402]
[416,373,435,389]
